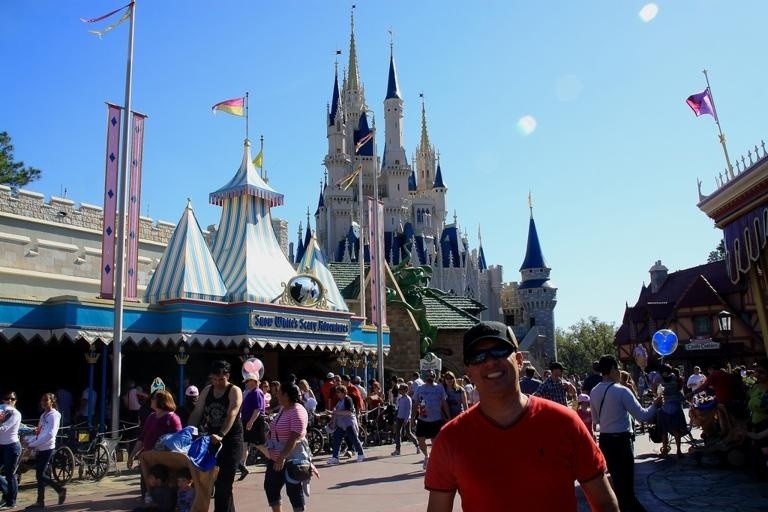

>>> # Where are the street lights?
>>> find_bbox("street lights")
[717,308,735,375]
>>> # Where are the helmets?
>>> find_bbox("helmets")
[578,393,590,402]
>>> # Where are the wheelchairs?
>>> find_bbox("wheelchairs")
[51,422,113,484]
[246,411,351,465]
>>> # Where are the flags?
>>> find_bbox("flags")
[253,149,262,169]
[212,97,244,119]
[686,86,715,116]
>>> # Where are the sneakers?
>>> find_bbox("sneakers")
[423,458,428,469]
[391,449,401,455]
[327,457,340,464]
[357,454,364,461]
[346,451,352,458]
[59,489,66,503]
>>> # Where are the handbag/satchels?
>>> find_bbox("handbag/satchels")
[286,462,313,481]
[647,424,662,443]
[324,414,337,433]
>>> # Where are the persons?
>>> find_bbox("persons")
[1,322,768,511]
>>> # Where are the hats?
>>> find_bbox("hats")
[550,362,566,370]
[186,385,199,397]
[463,320,518,355]
[242,373,258,382]
[398,383,408,390]
[327,372,361,382]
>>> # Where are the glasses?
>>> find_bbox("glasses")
[469,345,513,364]
[5,396,16,401]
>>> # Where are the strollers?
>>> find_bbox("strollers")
[138,427,225,511]
[658,422,704,455]
[359,404,395,446]
